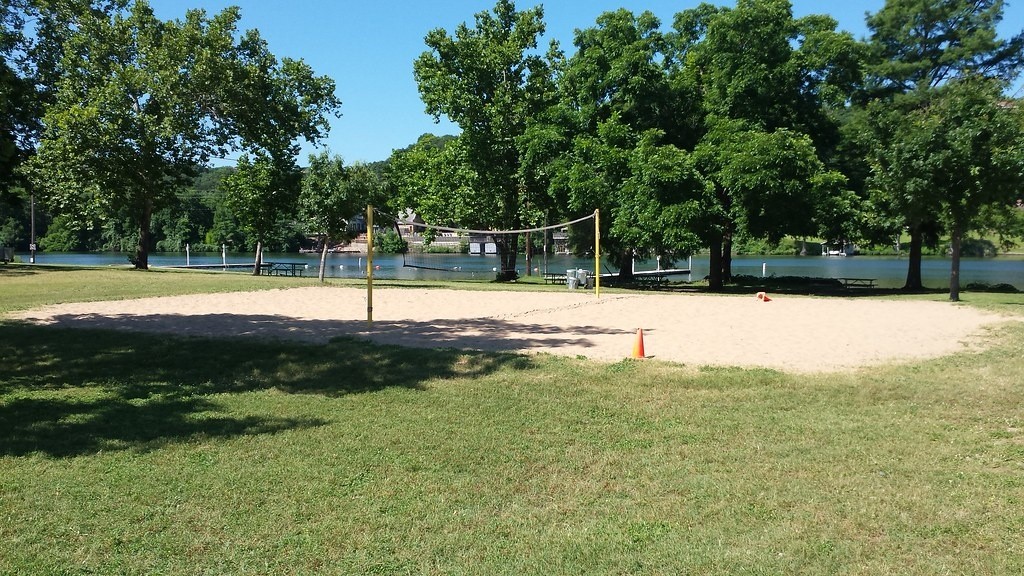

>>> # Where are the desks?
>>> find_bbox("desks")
[261,262,308,276]
[838,277,878,289]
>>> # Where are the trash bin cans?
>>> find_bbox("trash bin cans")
[569,276,580,289]
[566,269,589,285]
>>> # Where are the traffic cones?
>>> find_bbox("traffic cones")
[631,329,644,358]
[757,292,772,302]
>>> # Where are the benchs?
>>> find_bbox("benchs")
[261,269,271,270]
[843,284,878,287]
[273,269,303,271]
[543,272,567,285]
